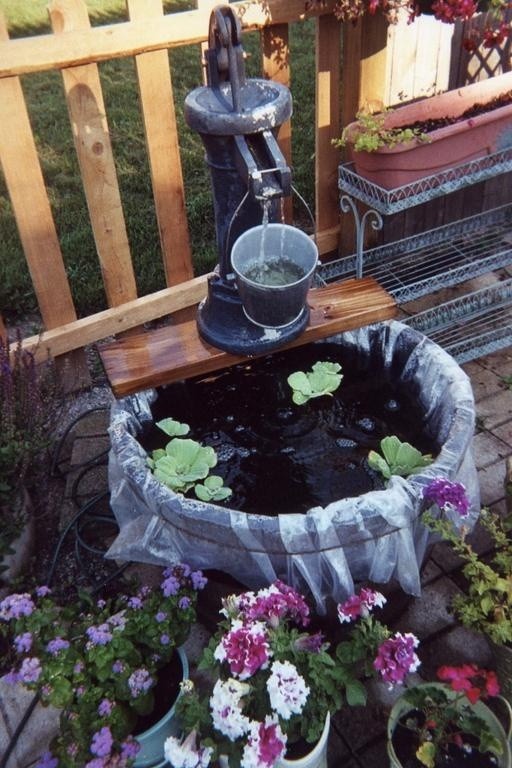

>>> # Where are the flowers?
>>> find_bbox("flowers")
[405,659,503,767]
[0,309,81,562]
[163,578,422,768]
[1,562,209,768]
[418,478,512,645]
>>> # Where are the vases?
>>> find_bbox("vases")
[1,486,37,590]
[486,632,512,709]
[132,645,189,767]
[480,692,512,743]
[385,682,512,768]
[278,710,330,768]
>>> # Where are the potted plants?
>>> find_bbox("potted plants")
[330,71,512,202]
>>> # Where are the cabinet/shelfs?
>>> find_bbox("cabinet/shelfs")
[314,146,512,365]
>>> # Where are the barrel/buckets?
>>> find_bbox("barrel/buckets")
[223,177,319,331]
